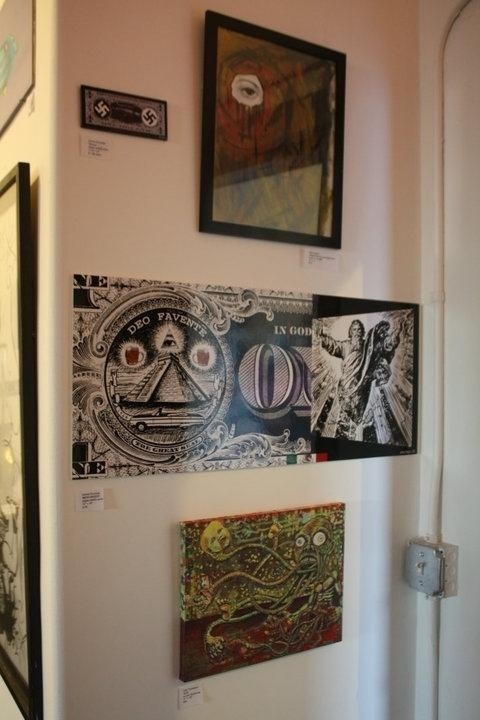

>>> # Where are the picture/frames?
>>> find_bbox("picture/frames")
[0,161,44,720]
[1,0,34,135]
[180,503,344,684]
[70,271,418,481]
[197,8,350,249]
[80,84,169,145]
